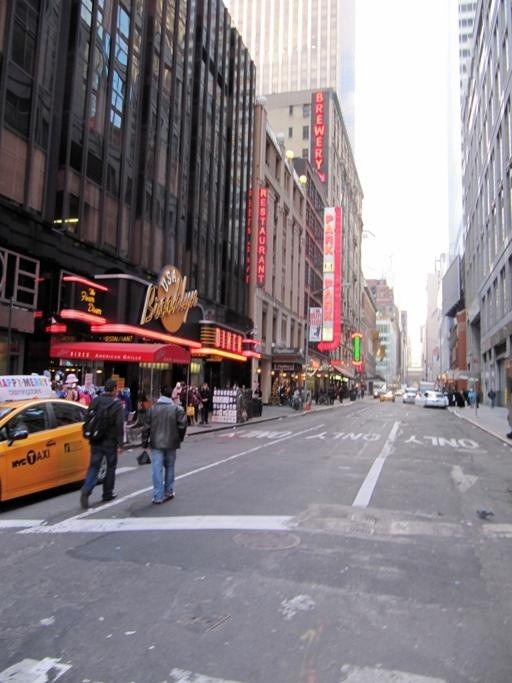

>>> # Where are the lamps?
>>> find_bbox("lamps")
[300,175,307,189]
[286,150,295,164]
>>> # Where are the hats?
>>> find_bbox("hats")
[65,373,78,383]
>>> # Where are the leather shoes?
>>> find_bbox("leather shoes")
[102,492,117,500]
[81,487,90,508]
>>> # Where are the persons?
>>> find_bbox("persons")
[449,388,496,408]
[141,384,187,503]
[80,379,124,508]
[276,382,363,407]
[171,382,212,425]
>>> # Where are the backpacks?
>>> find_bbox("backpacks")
[83,398,120,440]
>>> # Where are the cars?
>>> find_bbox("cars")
[374,381,450,408]
[1,400,120,503]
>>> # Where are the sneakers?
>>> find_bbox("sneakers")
[152,491,173,504]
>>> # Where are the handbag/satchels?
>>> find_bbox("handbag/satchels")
[187,406,196,416]
